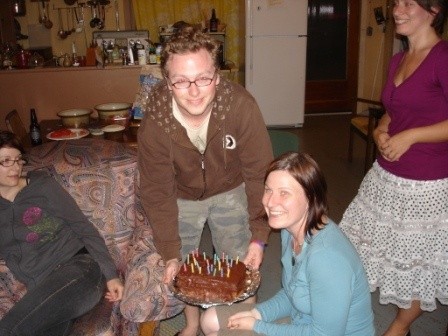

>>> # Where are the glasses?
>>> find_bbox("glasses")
[0,158,27,167]
[164,68,218,89]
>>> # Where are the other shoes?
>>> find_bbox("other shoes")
[404,329,411,336]
[174,332,180,336]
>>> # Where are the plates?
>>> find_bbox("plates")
[172,260,261,306]
[45,128,90,140]
[101,125,126,133]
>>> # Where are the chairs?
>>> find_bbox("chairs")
[349,98,386,176]
[5,109,28,141]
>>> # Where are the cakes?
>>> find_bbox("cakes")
[173,252,247,302]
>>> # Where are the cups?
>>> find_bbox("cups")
[56,55,65,66]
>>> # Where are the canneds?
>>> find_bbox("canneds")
[138,49,147,65]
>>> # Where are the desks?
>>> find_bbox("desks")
[22,119,138,150]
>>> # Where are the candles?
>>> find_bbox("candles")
[183,247,240,278]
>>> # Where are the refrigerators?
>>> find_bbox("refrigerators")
[244,0,309,128]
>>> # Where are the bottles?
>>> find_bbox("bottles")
[210,8,218,32]
[29,108,42,146]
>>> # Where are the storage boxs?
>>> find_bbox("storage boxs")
[28,46,57,67]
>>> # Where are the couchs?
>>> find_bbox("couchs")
[0,139,186,336]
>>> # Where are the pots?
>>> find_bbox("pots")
[93,102,133,125]
[55,107,94,128]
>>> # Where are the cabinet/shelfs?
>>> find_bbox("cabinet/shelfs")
[0,65,163,133]
